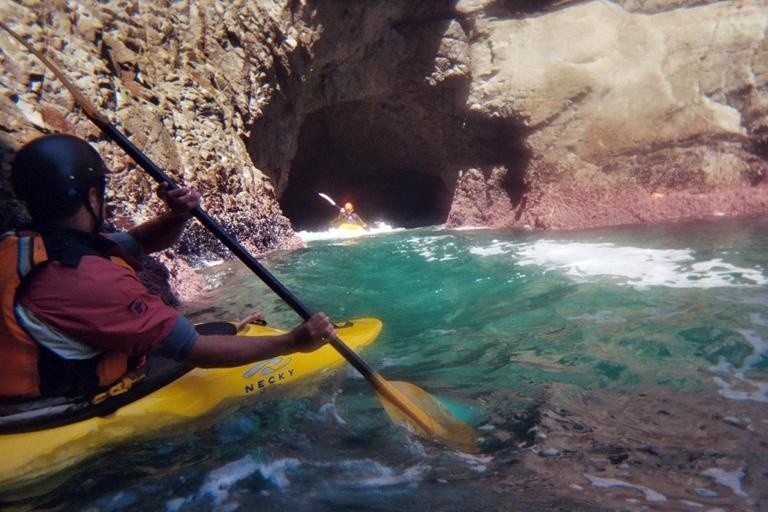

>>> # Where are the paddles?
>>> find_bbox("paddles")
[320,191,357,227]
[0,19,478,453]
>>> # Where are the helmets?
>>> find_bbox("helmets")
[13,134,107,217]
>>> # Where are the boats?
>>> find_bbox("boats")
[324,215,368,234]
[0,301,382,492]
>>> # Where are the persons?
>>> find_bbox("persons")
[332,202,368,229]
[0,133,336,404]
